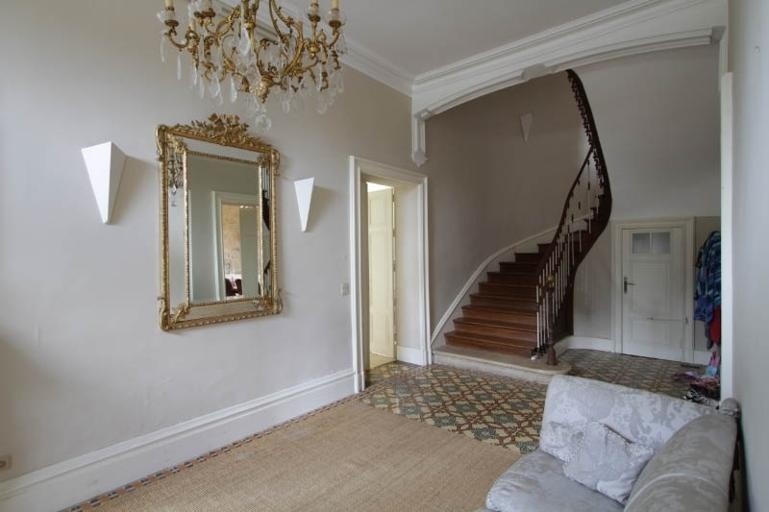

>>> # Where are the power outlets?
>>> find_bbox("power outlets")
[0,455,12,471]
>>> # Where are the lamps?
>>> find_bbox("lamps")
[157,0,349,131]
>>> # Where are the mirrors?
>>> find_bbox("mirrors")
[157,112,283,330]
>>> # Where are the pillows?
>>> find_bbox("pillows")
[560,423,657,506]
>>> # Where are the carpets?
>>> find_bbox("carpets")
[84,396,524,512]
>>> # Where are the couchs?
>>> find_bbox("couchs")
[476,374,737,512]
